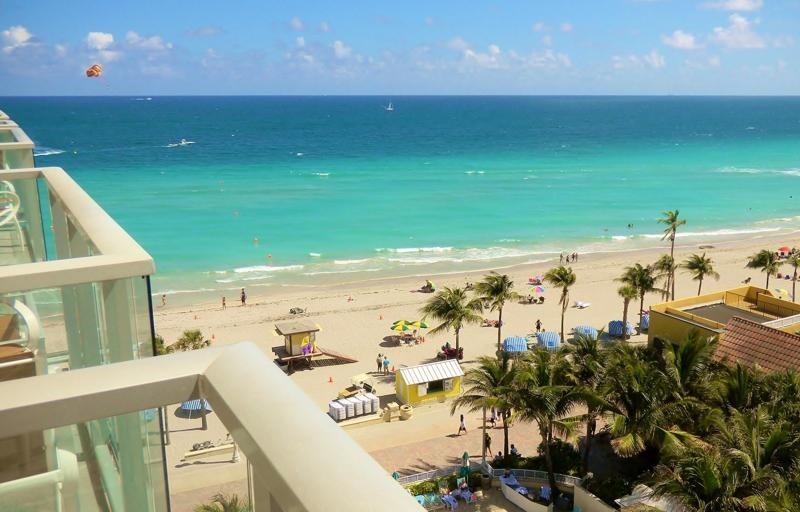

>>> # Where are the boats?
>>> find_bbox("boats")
[181,138,196,145]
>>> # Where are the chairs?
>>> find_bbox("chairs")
[437,480,458,512]
[497,473,534,496]
[538,484,552,505]
[457,475,478,506]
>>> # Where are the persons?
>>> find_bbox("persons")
[162,294,166,305]
[383,356,391,376]
[527,295,545,304]
[535,319,542,331]
[558,252,579,263]
[377,353,383,374]
[222,297,226,306]
[484,405,518,464]
[437,342,463,359]
[458,414,468,435]
[241,287,247,302]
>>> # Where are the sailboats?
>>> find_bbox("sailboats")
[387,97,394,112]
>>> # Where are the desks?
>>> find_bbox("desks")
[440,346,462,360]
[402,332,414,343]
[380,335,399,347]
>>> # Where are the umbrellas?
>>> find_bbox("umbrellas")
[528,274,545,295]
[390,319,427,337]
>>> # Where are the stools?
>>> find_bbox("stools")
[0,149,94,512]
